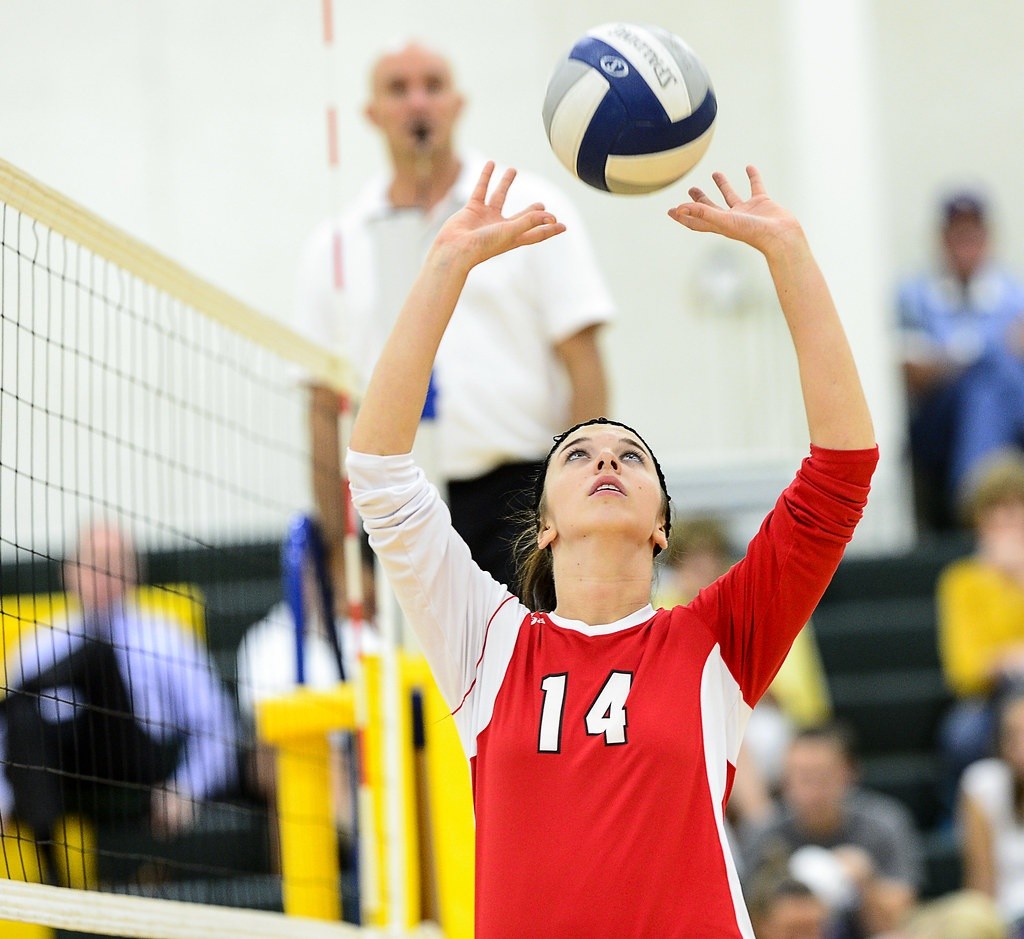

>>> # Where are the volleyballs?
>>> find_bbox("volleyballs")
[541,17,722,196]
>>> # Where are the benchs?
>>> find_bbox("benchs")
[1,540,1024,938]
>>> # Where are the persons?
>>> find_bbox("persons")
[0,40,1024,939]
